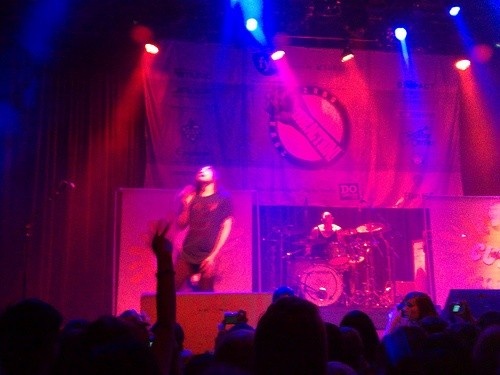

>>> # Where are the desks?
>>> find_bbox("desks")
[140,291,273,356]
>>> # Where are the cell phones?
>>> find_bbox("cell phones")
[451,304,461,312]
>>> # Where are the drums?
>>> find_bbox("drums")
[329,255,348,271]
[334,242,371,265]
[287,256,326,285]
[299,264,344,307]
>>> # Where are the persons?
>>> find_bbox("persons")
[0,227,500,375]
[306,211,341,257]
[173,162,234,293]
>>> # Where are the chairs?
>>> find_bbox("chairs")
[340,40,356,62]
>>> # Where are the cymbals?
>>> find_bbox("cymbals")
[357,222,384,233]
[336,230,361,241]
